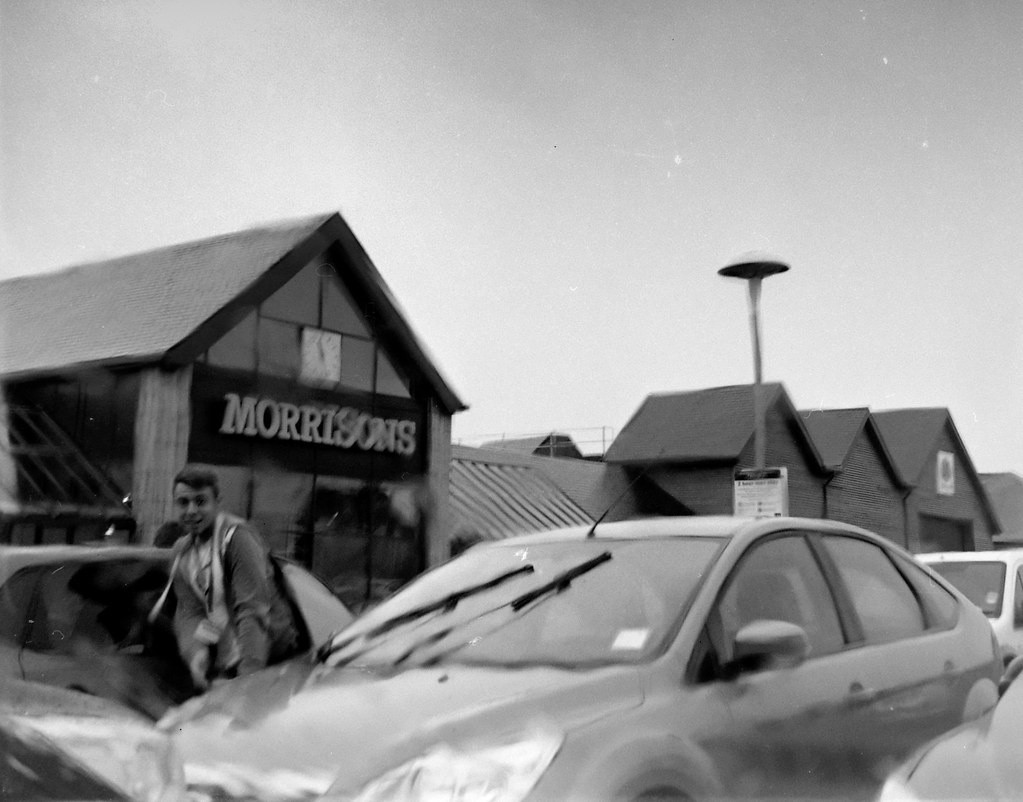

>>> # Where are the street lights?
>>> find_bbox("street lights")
[715,256,790,469]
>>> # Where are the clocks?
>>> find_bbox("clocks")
[295,324,343,392]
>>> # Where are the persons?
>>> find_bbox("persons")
[162,462,300,694]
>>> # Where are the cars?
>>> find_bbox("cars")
[1,543,359,723]
[910,545,1022,679]
[0,514,1022,802]
